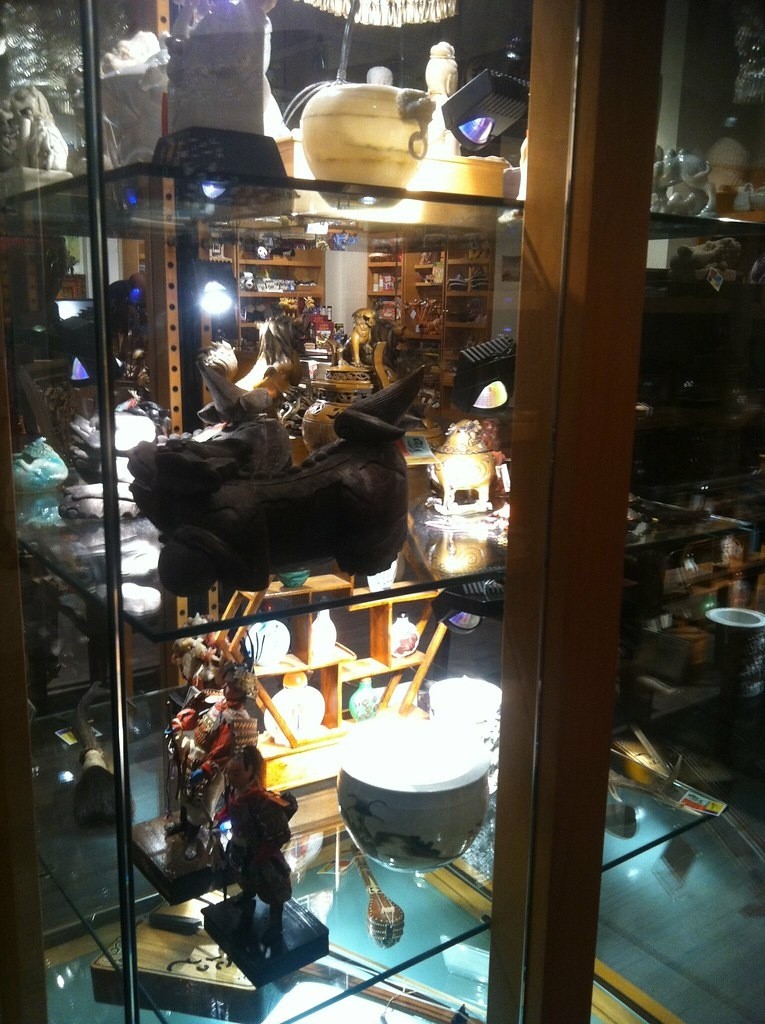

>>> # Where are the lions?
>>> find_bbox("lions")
[342,308,377,368]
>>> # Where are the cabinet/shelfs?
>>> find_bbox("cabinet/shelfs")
[0,0,765,1024]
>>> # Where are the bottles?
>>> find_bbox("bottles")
[349,677,378,722]
[311,609,336,659]
[264,672,325,747]
[389,609,422,659]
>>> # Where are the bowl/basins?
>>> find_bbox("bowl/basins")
[335,745,489,872]
[278,570,310,588]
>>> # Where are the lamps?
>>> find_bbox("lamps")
[301,0,456,25]
[440,65,529,150]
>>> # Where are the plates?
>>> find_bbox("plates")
[244,618,290,664]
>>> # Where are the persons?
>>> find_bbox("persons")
[60,273,149,381]
[163,631,259,860]
[214,742,292,934]
